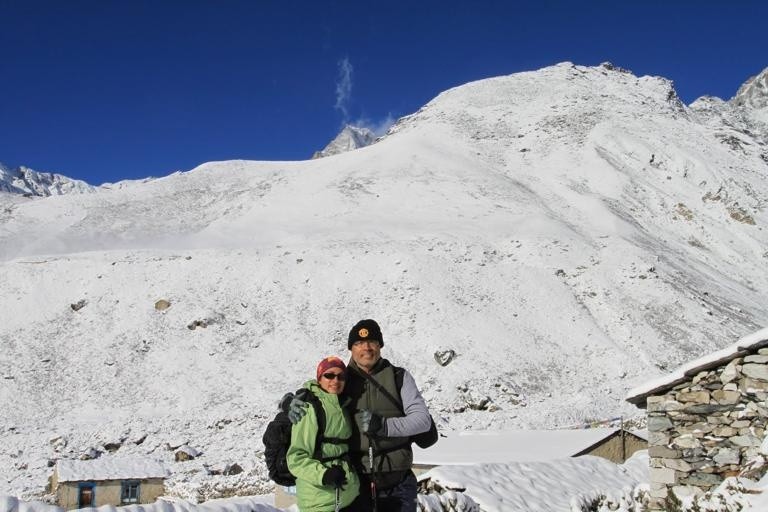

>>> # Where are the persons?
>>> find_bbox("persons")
[286,357,360,512]
[289,319,432,512]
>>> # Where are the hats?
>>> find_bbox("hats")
[348,319,384,350]
[317,357,347,381]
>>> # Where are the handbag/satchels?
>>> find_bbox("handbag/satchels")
[410,415,438,448]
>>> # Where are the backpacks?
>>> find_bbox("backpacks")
[263,389,326,486]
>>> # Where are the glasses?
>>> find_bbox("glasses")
[353,340,380,346]
[322,373,345,380]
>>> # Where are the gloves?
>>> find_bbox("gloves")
[322,464,348,489]
[277,391,309,424]
[358,409,388,439]
